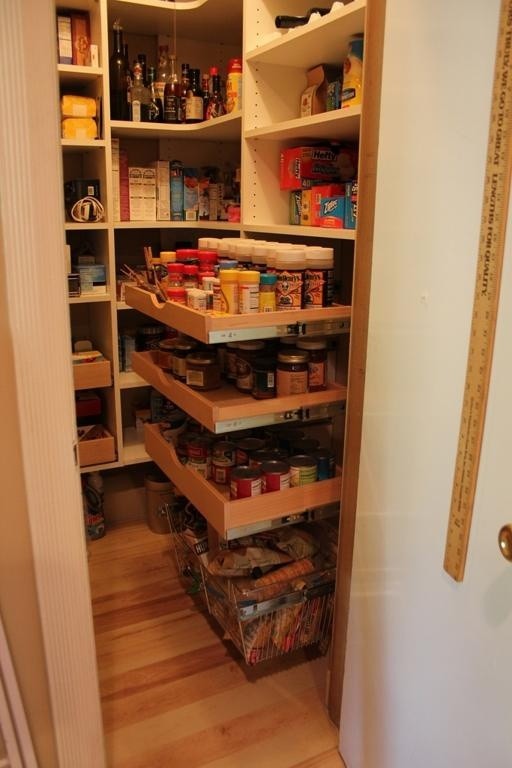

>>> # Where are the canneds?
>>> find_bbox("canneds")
[159,335,327,400]
[148,238,337,315]
[157,409,336,500]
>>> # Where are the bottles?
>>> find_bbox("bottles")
[157,235,335,317]
[275,8,329,30]
[109,15,242,124]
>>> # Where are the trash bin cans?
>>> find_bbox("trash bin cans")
[144,473,178,535]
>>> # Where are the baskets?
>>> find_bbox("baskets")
[164,501,335,666]
